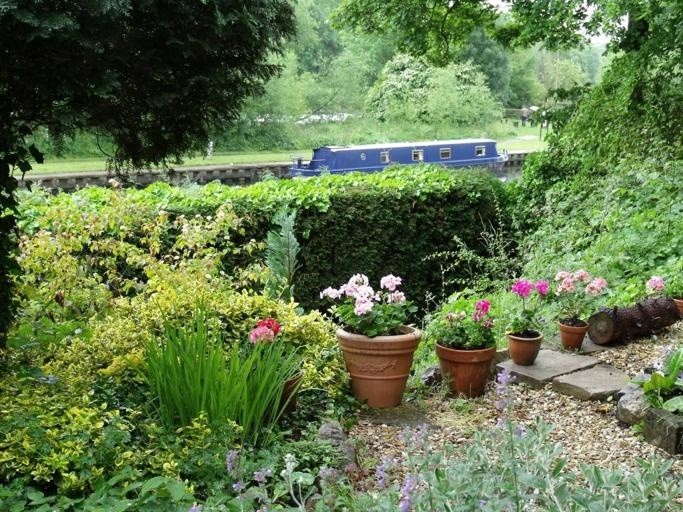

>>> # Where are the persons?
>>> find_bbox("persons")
[520,104,550,130]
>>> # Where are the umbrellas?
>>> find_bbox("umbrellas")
[531,105,541,113]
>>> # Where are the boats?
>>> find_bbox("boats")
[288,137,507,187]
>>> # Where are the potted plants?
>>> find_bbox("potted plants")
[668,262,682,321]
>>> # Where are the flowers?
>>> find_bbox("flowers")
[436,300,497,347]
[553,269,607,311]
[601,273,661,308]
[509,278,554,334]
[321,272,417,337]
[246,316,282,346]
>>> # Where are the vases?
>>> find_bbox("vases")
[509,329,542,367]
[556,318,588,352]
[276,366,306,415]
[434,336,498,394]
[334,325,422,409]
[584,293,679,346]
